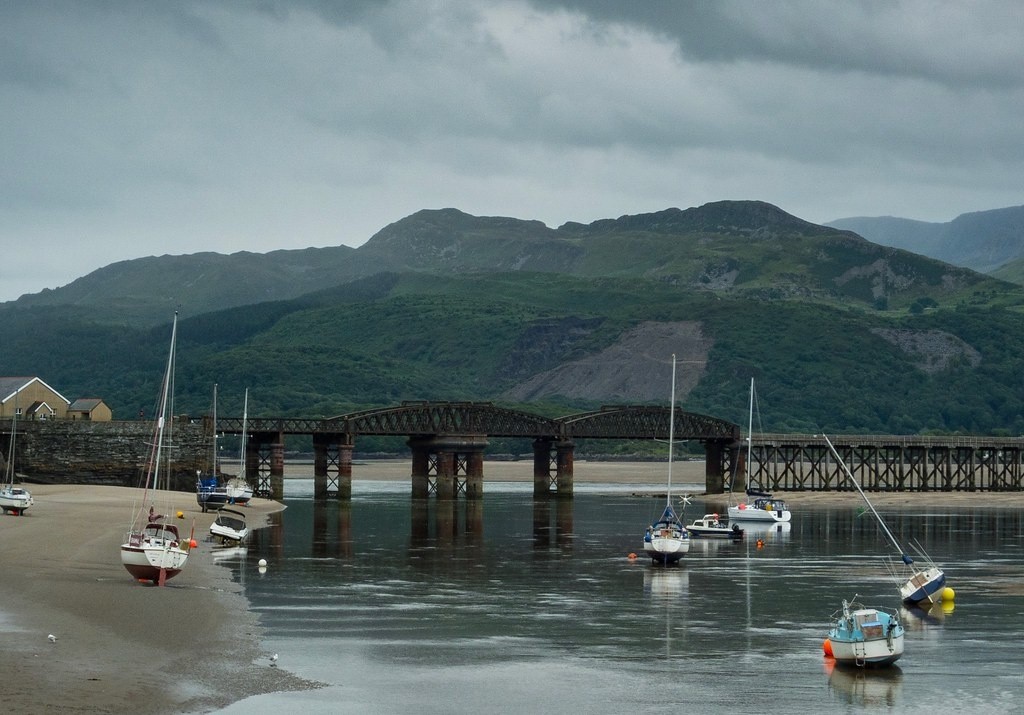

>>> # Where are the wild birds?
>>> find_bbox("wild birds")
[48,632,58,642]
[269,654,278,663]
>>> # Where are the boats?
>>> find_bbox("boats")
[746,489,772,497]
[685,513,744,540]
[823,597,905,669]
[209,507,250,546]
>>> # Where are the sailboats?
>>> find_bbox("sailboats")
[821,432,946,606]
[727,377,792,522]
[196,383,227,511]
[120,310,196,586]
[226,386,254,505]
[0,389,34,516]
[643,351,690,567]
